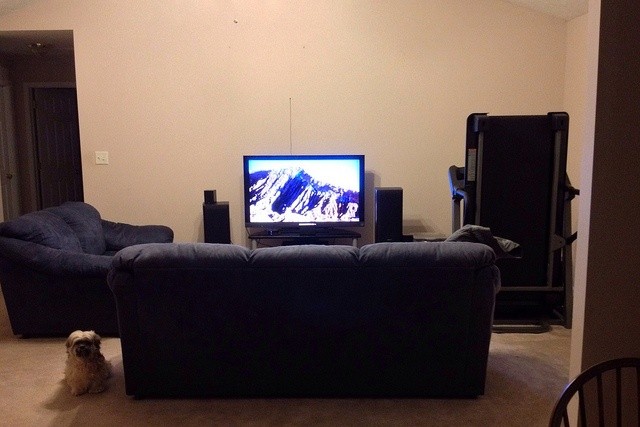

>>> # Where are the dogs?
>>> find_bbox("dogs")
[62,329,117,397]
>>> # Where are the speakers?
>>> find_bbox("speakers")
[202,200,231,243]
[374,185,403,241]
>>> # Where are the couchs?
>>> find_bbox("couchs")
[0,201,174,337]
[104,241,505,402]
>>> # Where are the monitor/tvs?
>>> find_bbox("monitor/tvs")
[243,154,365,233]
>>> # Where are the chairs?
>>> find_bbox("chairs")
[548,353,639,427]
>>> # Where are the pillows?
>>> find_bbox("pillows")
[440,224,519,266]
